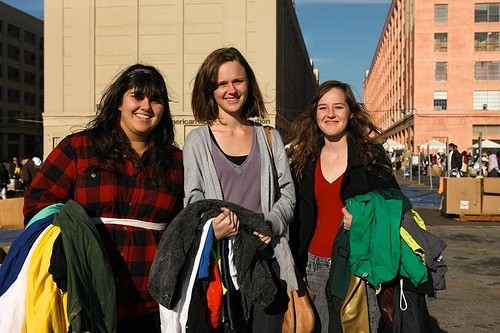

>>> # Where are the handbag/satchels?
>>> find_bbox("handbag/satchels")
[281,266,317,333]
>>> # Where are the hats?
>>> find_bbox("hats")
[32,156,41,166]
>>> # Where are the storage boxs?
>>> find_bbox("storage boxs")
[442,177,482,214]
[482,195,500,216]
[481,177,500,194]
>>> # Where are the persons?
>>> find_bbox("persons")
[386,143,500,178]
[181,47,297,333]
[0,156,42,199]
[289,80,401,333]
[21,64,184,333]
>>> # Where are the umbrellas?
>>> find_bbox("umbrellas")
[419,139,446,155]
[382,139,405,151]
[468,140,500,148]
